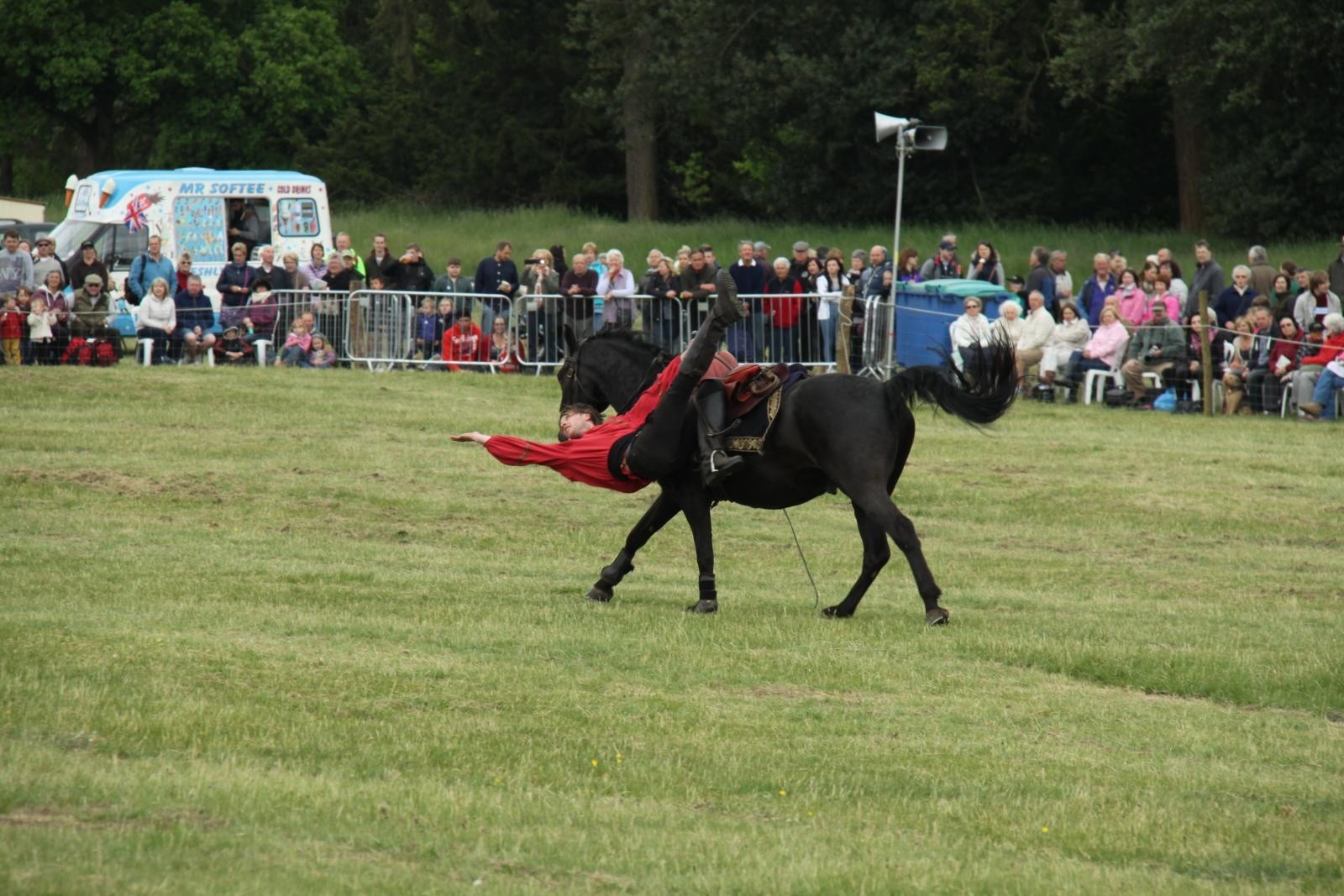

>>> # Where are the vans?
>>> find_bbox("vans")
[36,167,335,320]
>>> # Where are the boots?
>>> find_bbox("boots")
[698,390,745,489]
[678,269,746,382]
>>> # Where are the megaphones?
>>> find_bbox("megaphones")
[903,125,947,150]
[874,111,920,143]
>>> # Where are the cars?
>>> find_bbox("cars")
[0,221,60,261]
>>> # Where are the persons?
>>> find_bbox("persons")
[450,268,753,491]
[0,229,1344,422]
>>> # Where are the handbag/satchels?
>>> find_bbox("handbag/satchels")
[125,254,146,305]
[61,336,116,366]
[109,308,137,336]
[321,299,340,315]
[617,309,631,326]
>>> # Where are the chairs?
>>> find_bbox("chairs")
[251,310,283,367]
[131,306,154,366]
[1065,335,1132,405]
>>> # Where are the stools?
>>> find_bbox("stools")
[1184,378,1224,415]
[1280,382,1305,418]
[1127,370,1161,391]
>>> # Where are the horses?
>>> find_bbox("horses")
[556,320,1037,628]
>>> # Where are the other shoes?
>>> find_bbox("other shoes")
[1032,385,1323,416]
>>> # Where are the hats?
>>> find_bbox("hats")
[1151,301,1166,311]
[793,241,808,252]
[253,278,273,291]
[20,239,31,248]
[342,249,354,258]
[81,241,94,248]
[35,232,52,245]
[1309,323,1326,331]
[1007,276,1025,284]
[456,308,469,319]
[941,243,957,251]
[85,273,103,283]
[852,249,868,265]
[755,242,771,251]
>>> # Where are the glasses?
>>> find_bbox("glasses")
[967,306,978,308]
[181,261,190,265]
[1281,323,1293,328]
[38,244,50,246]
[89,283,99,287]
